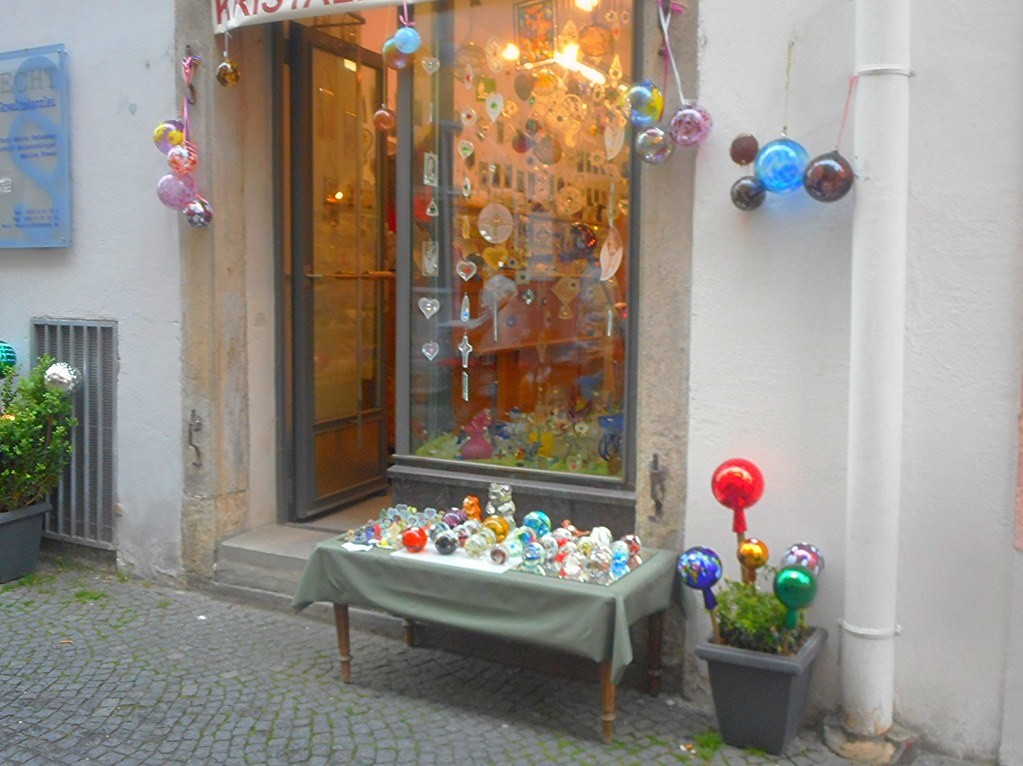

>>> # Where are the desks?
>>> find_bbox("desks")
[290,516,678,744]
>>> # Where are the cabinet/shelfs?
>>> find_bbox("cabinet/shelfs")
[385,200,624,441]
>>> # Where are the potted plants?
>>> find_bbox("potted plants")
[695,579,829,755]
[0,354,79,587]
